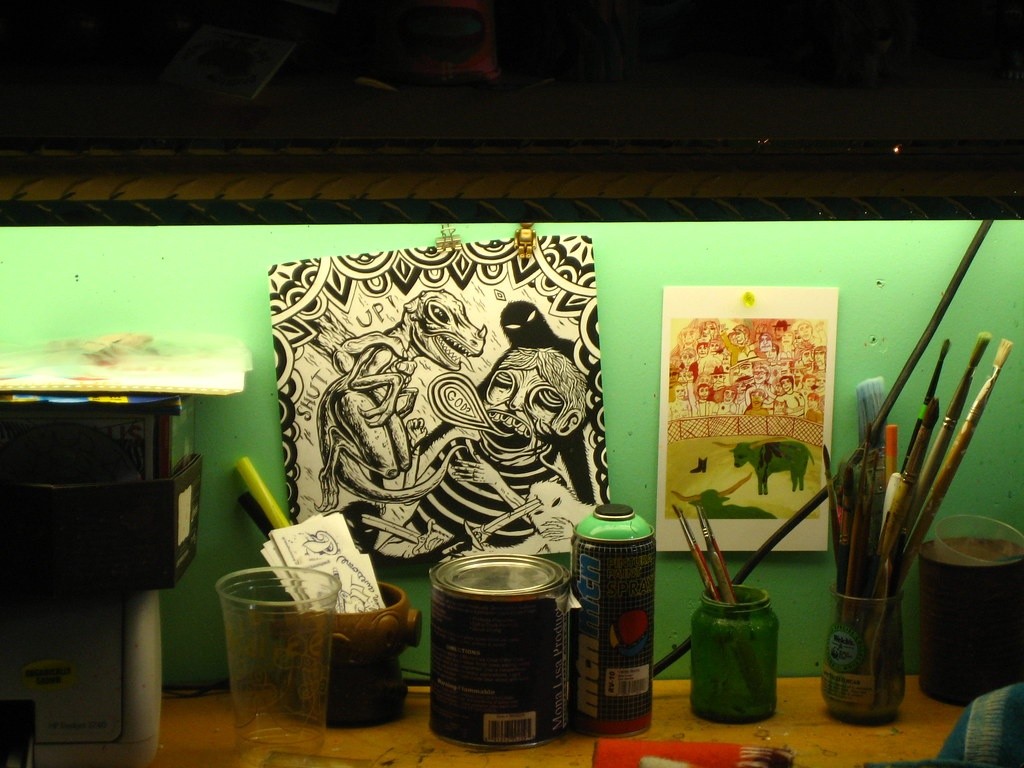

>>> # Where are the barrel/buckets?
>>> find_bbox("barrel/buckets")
[916,537,1024,707]
[427,553,572,751]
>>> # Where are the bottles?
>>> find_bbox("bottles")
[820,582,906,719]
[689,584,779,723]
[568,504,657,738]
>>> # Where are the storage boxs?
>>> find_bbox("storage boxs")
[0,451,201,604]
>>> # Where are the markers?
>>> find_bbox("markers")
[234,456,292,541]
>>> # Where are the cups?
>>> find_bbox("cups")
[214,567,341,767]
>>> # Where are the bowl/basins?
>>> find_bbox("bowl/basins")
[934,514,1024,568]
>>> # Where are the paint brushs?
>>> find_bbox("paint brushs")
[672,500,739,603]
[820,332,1014,599]
